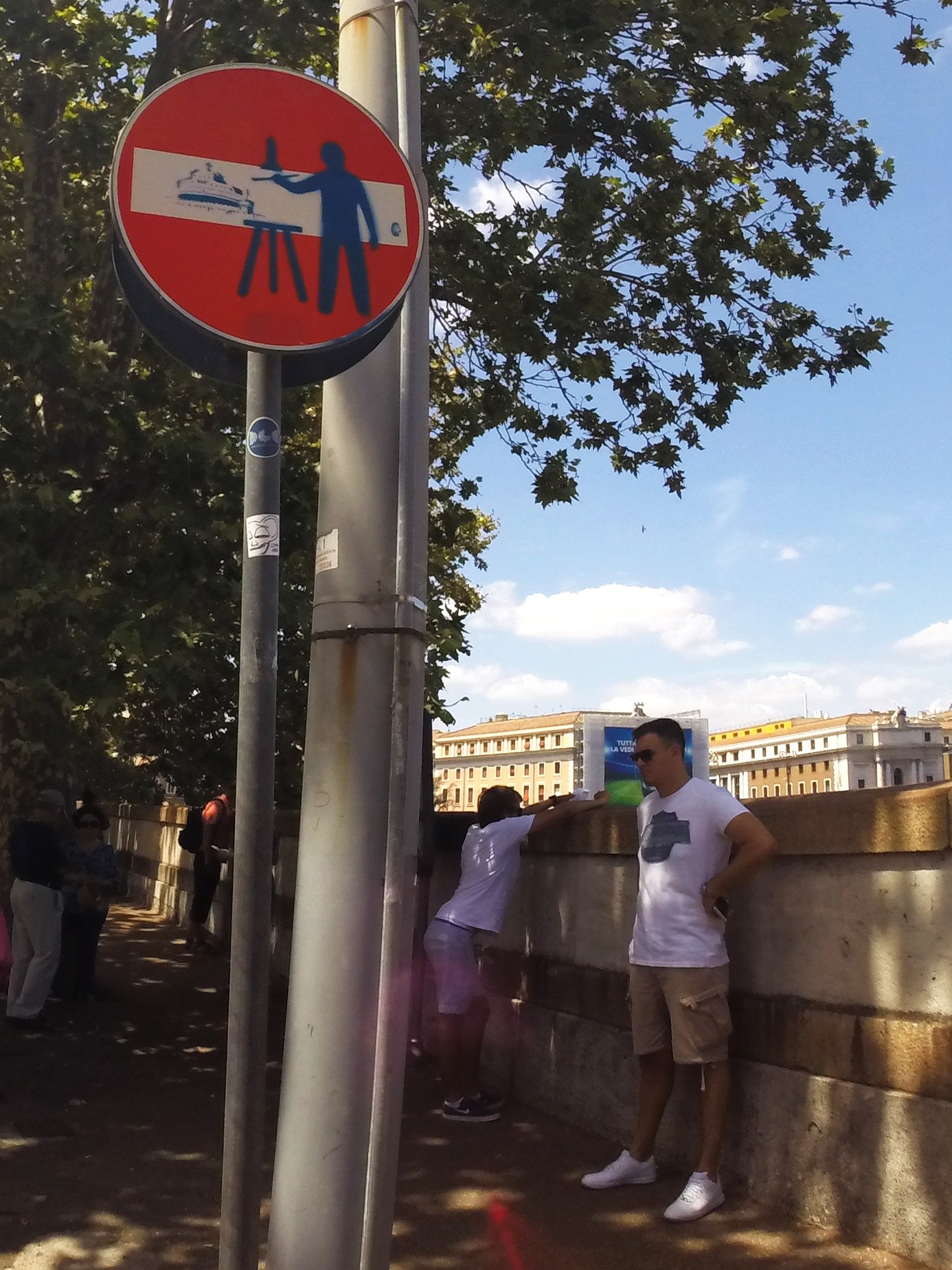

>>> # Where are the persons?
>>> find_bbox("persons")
[185,772,237,954]
[580,719,776,1221]
[0,789,119,1032]
[424,785,611,1123]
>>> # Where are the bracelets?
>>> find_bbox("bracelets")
[548,794,557,807]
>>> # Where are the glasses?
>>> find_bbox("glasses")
[630,745,672,762]
[78,820,100,829]
[514,807,522,817]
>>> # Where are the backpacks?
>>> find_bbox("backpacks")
[178,798,225,854]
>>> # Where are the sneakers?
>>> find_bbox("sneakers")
[473,1089,507,1108]
[443,1095,501,1121]
[663,1171,725,1221]
[580,1149,658,1189]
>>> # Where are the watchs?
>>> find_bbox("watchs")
[698,883,720,899]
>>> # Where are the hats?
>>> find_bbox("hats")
[39,789,70,820]
[72,806,110,830]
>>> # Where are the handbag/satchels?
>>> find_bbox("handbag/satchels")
[77,876,109,911]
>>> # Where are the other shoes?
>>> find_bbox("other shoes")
[15,1015,53,1031]
